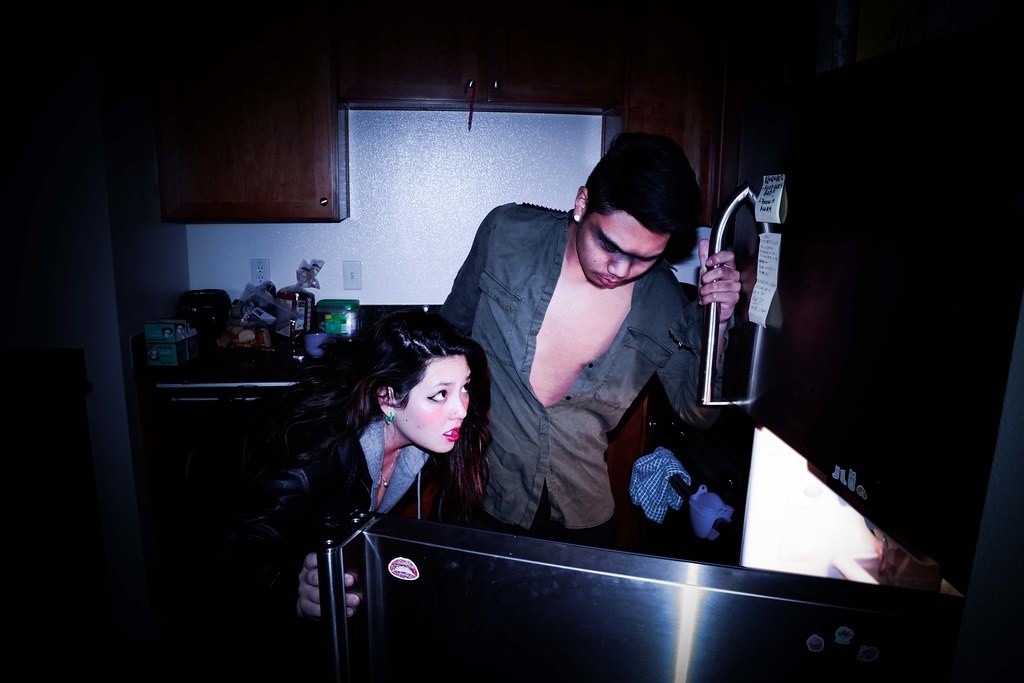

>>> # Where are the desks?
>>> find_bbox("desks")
[157,340,306,489]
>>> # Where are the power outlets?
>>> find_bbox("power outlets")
[251,258,269,287]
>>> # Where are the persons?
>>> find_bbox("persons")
[183,308,493,683]
[440,130,741,544]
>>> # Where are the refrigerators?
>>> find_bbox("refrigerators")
[320,1,1023,683]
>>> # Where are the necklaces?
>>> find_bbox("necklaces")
[379,445,398,487]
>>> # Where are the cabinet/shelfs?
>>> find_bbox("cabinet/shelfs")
[146,0,746,225]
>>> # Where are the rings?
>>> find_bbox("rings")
[303,567,312,585]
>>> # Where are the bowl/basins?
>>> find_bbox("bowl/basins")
[305,334,328,358]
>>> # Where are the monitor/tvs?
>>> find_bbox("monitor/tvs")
[737,424,967,598]
